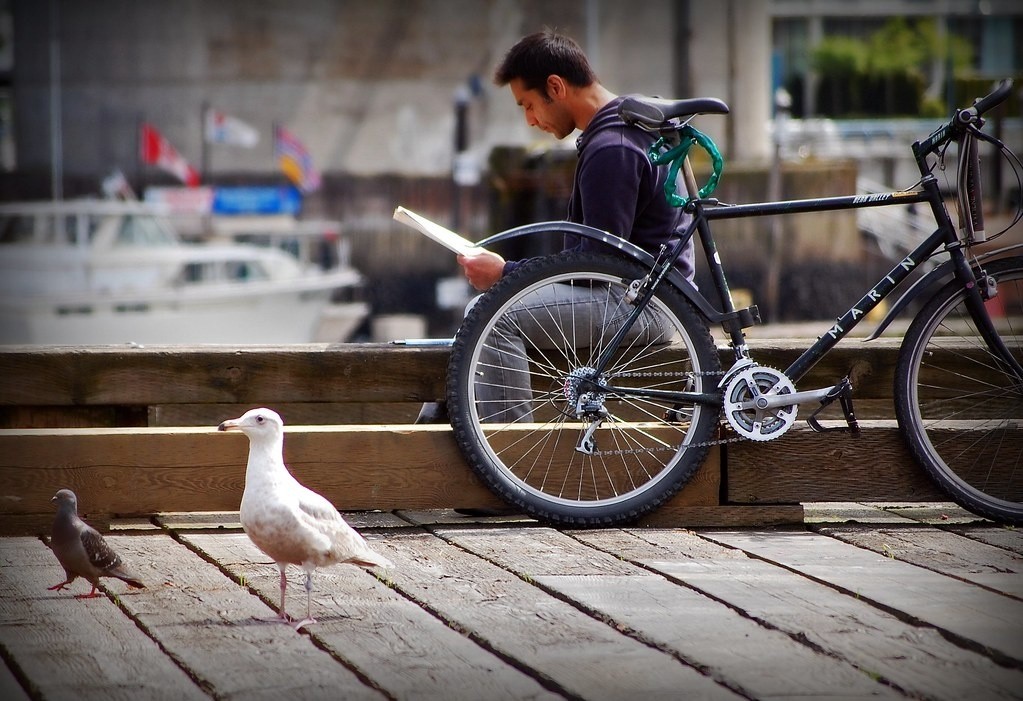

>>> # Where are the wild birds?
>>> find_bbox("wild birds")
[218,406,395,632]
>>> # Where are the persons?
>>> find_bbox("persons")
[412,30,698,517]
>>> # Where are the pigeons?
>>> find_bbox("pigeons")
[46,487,148,599]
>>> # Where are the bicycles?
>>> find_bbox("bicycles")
[445,79,1023,528]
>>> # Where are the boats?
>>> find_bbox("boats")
[0,200,371,344]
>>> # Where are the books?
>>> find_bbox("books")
[392,205,505,264]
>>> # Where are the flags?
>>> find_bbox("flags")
[203,107,258,150]
[276,126,323,195]
[142,122,200,190]
